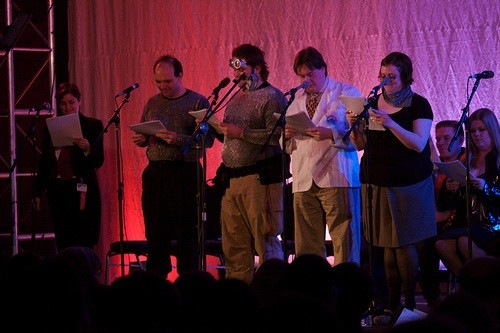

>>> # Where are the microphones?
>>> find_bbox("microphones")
[283,81,309,96]
[207,77,231,100]
[370,77,391,94]
[469,70,495,78]
[116,82,139,97]
[29,102,50,112]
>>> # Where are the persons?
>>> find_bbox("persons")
[431,120,467,237]
[132,55,215,279]
[195,44,288,284]
[35,83,105,250]
[435,109,500,297]
[279,46,361,267]
[0,247,500,333]
[346,51,438,325]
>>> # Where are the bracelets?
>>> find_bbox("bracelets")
[349,131,360,140]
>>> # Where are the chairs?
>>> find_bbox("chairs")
[105,179,334,286]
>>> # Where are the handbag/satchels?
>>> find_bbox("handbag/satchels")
[257,152,292,184]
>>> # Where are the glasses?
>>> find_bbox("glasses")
[229,58,246,68]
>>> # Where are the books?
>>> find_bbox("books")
[273,112,317,135]
[188,109,220,127]
[436,160,475,187]
[130,120,167,137]
[339,96,386,131]
[46,112,83,147]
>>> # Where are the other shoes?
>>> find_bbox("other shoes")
[373,309,393,325]
